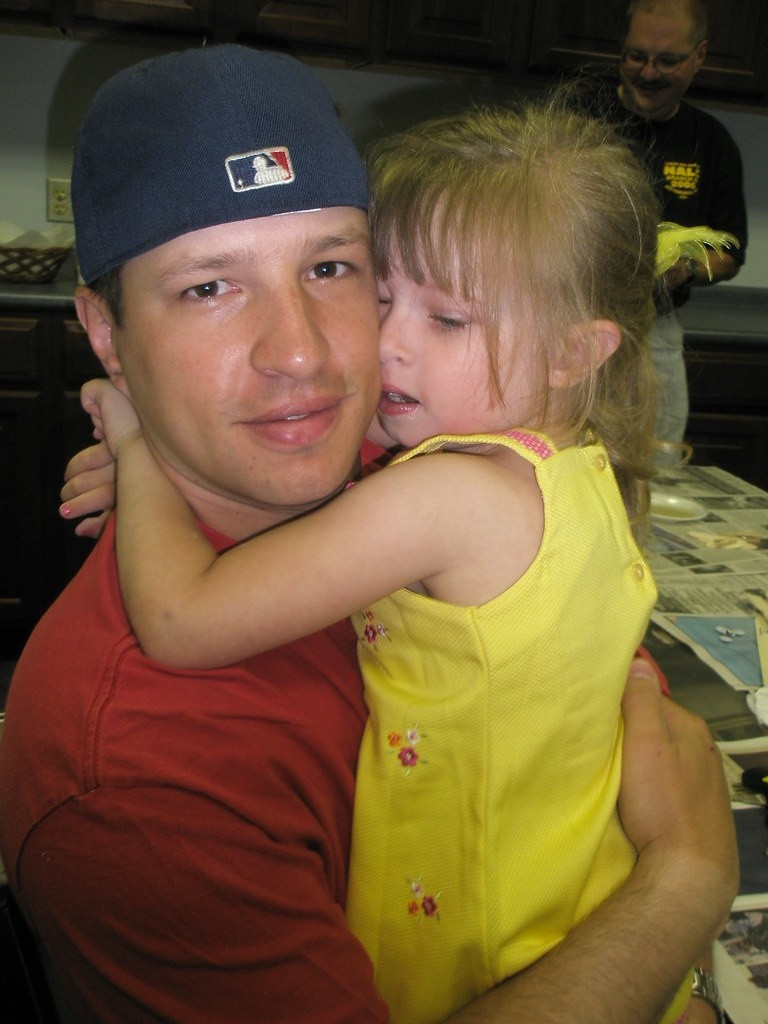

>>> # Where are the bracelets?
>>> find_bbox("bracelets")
[691,968,726,1024]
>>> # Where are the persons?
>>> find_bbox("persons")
[1,45,738,1024]
[550,0,748,469]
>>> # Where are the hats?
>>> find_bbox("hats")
[69,43,369,286]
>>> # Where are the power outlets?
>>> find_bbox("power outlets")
[44,176,77,225]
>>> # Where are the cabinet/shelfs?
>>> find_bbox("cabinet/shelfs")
[0,0,768,702]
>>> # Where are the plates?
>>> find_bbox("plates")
[650,493,708,522]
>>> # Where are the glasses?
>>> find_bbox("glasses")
[620,39,700,75]
